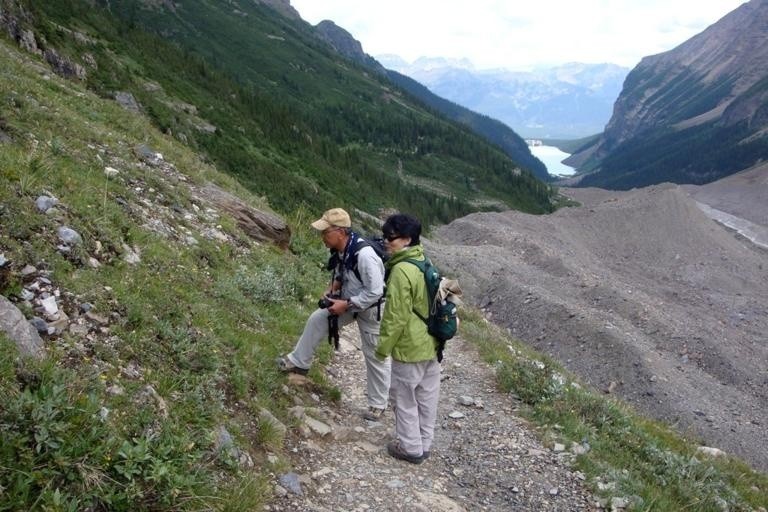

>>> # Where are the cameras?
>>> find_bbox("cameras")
[317,293,339,309]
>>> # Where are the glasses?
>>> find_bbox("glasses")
[383,234,401,242]
[321,227,341,237]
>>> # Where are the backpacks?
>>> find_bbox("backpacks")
[404,253,457,339]
[326,232,390,284]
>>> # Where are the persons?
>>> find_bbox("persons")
[277,207,392,421]
[375,214,441,464]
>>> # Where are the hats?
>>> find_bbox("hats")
[310,207,352,231]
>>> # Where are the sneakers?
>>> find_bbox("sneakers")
[279,358,309,376]
[387,442,431,465]
[364,405,386,421]
